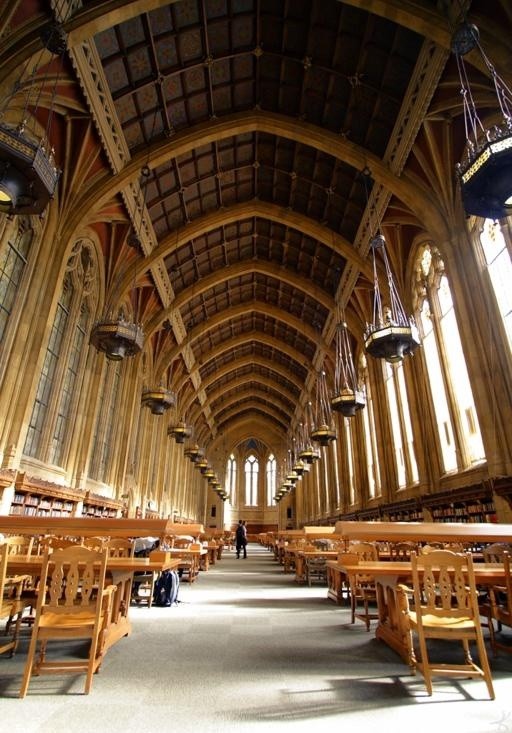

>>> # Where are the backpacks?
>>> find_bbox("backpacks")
[236,524,244,538]
[152,567,183,609]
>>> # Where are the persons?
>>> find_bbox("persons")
[235,519,247,559]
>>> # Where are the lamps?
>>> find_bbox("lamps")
[141,306,176,416]
[1,0,83,226]
[355,204,422,365]
[449,17,512,221]
[166,390,193,445]
[182,439,231,501]
[328,317,368,419]
[272,369,338,507]
[85,98,167,362]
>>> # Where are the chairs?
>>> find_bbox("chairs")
[492,550,512,635]
[1,503,238,652]
[260,506,511,633]
[1,543,28,661]
[17,539,118,698]
[392,547,497,701]
